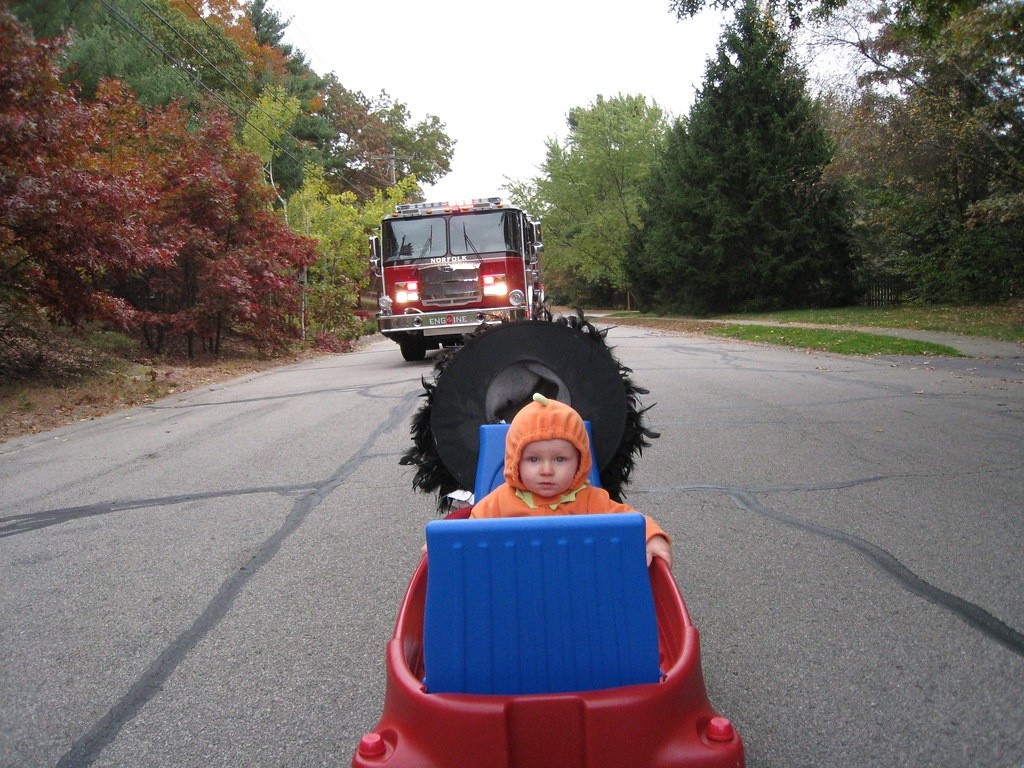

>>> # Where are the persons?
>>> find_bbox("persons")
[420,393,673,570]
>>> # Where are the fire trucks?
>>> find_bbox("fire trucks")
[367,195,548,363]
